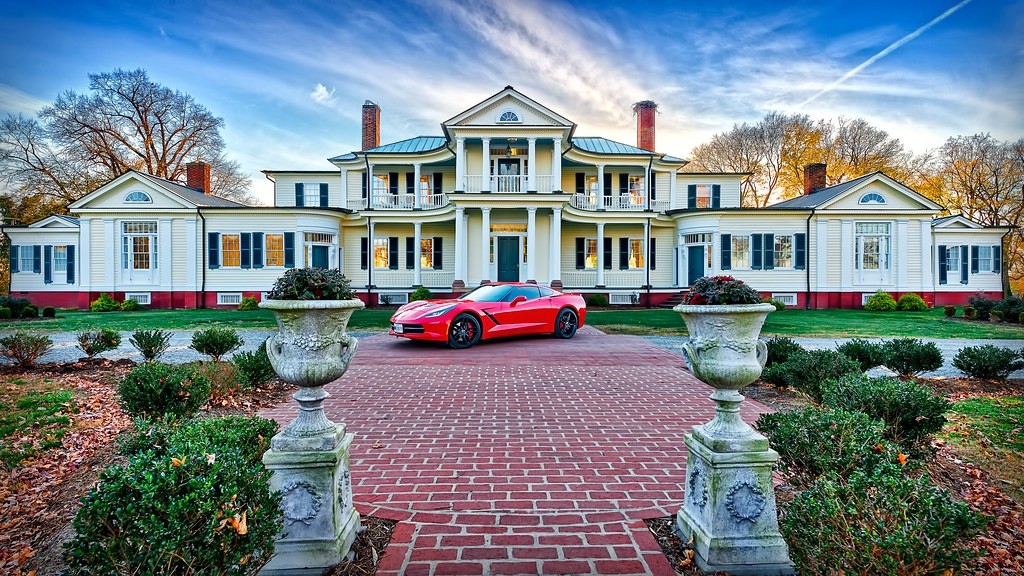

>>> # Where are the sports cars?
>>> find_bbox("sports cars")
[388,282,586,350]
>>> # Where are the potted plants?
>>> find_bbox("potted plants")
[586,296,608,312]
[257,266,366,441]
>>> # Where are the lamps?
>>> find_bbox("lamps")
[505,140,512,158]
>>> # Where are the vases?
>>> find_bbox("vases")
[672,302,778,452]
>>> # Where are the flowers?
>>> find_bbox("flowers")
[682,275,762,305]
